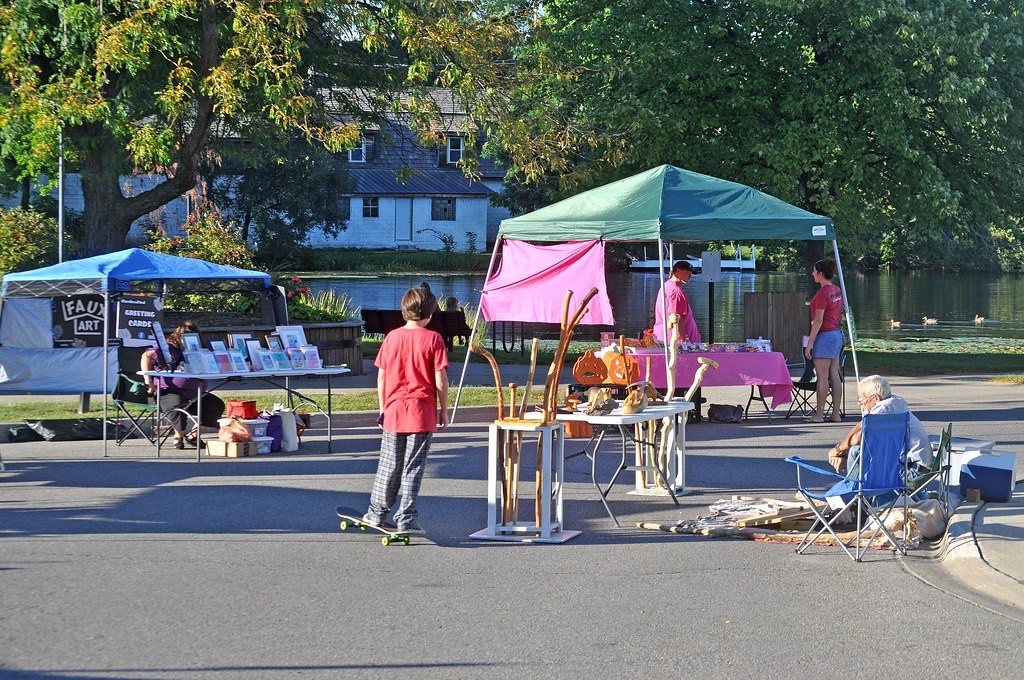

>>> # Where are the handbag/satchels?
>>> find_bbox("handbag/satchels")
[218,416,252,442]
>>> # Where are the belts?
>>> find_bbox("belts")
[909,462,931,474]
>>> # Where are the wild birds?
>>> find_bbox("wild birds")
[974,314,985,321]
[890,318,901,325]
[921,316,939,323]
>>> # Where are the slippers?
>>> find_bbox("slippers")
[822,417,841,423]
[803,417,824,423]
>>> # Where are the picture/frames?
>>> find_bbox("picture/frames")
[180,325,322,373]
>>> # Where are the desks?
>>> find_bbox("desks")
[135,369,351,463]
[515,400,693,526]
[592,346,794,423]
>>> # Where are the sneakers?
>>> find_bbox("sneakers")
[362,513,385,528]
[398,522,427,534]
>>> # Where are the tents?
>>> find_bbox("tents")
[1,248,273,456]
[449,165,863,427]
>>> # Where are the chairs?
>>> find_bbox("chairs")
[787,345,847,425]
[115,368,204,449]
[909,422,954,522]
[785,410,908,563]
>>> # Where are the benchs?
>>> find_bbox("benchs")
[361,306,478,352]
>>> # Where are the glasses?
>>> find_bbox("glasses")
[856,394,875,405]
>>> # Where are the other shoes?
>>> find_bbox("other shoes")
[183,434,206,450]
[174,434,184,450]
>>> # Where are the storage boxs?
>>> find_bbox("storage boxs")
[927,431,997,483]
[205,400,299,457]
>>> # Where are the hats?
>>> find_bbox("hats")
[674,261,696,271]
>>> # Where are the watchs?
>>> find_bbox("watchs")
[835,443,841,453]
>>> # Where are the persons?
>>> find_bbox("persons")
[653,261,701,347]
[141,319,225,450]
[813,375,935,533]
[805,259,844,423]
[363,287,449,533]
[418,282,469,347]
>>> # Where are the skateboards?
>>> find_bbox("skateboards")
[335,505,426,546]
